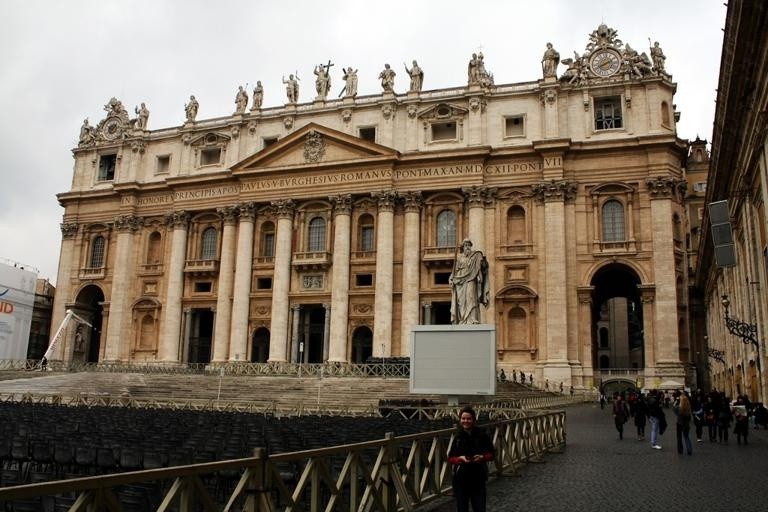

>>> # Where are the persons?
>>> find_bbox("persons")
[41,356,48,372]
[447,406,497,512]
[134,102,150,129]
[343,65,358,96]
[80,119,98,147]
[649,39,668,74]
[500,368,506,378]
[184,95,200,124]
[569,385,575,395]
[279,73,301,105]
[405,60,425,93]
[235,85,249,112]
[559,381,565,393]
[74,326,86,350]
[469,52,484,81]
[607,383,768,457]
[513,369,516,380]
[598,389,608,409]
[378,64,397,91]
[314,65,333,101]
[545,379,549,391]
[520,370,523,378]
[522,371,526,383]
[542,41,560,77]
[448,236,491,325]
[529,373,534,386]
[251,79,265,109]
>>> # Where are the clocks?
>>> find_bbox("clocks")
[588,45,622,80]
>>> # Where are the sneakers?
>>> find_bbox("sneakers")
[618,434,752,454]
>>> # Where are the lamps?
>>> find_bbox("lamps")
[695,350,713,373]
[721,293,758,346]
[704,335,726,368]
